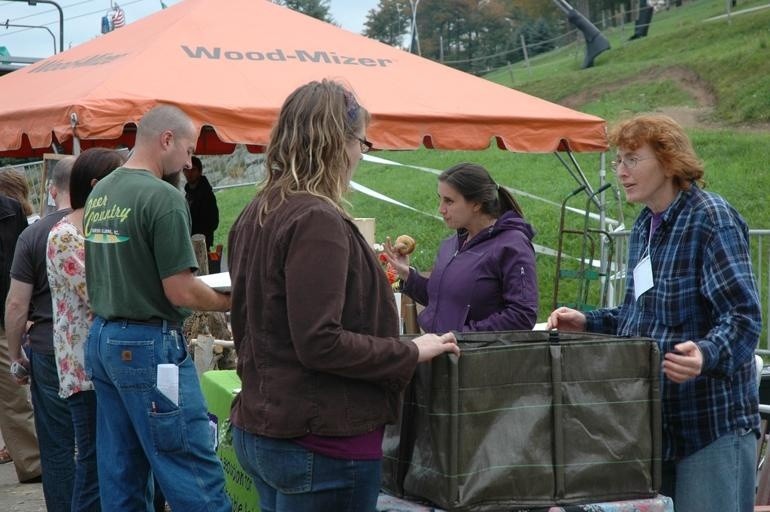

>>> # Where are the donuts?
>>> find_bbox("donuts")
[396,234,415,256]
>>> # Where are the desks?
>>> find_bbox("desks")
[200,369,260,512]
[376,492,674,512]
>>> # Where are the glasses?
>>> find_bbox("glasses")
[608,154,660,171]
[344,132,377,155]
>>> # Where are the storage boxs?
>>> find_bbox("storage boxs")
[380,328,661,511]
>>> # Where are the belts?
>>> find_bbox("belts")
[108,317,184,329]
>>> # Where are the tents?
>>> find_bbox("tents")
[0,1,610,158]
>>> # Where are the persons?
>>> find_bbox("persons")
[183,156,221,270]
[5,155,100,510]
[229,78,461,511]
[381,165,540,336]
[0,166,40,465]
[0,187,48,484]
[545,113,765,512]
[82,104,231,511]
[44,147,149,509]
